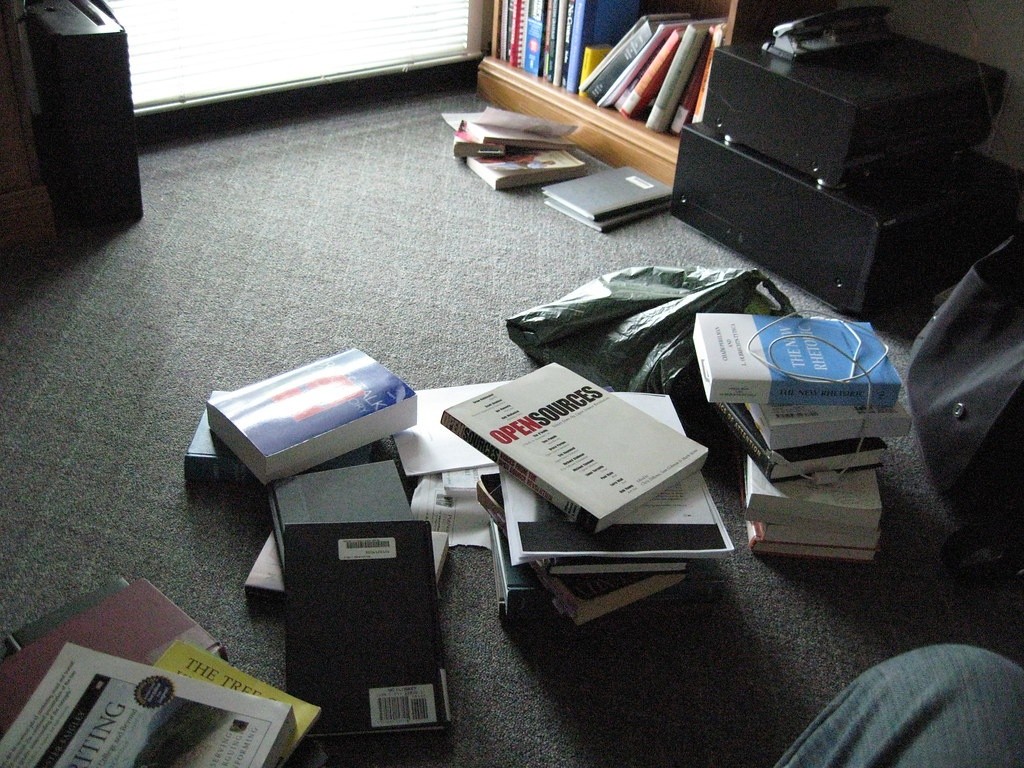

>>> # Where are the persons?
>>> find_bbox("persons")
[774,643,1024,768]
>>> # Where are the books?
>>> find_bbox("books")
[441,0,729,232]
[0,312,912,768]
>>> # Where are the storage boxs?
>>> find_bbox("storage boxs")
[703,33,1007,190]
[671,123,1024,316]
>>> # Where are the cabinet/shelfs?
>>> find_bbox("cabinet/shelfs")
[477,0,838,187]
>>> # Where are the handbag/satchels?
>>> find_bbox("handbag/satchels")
[905,234,1024,499]
